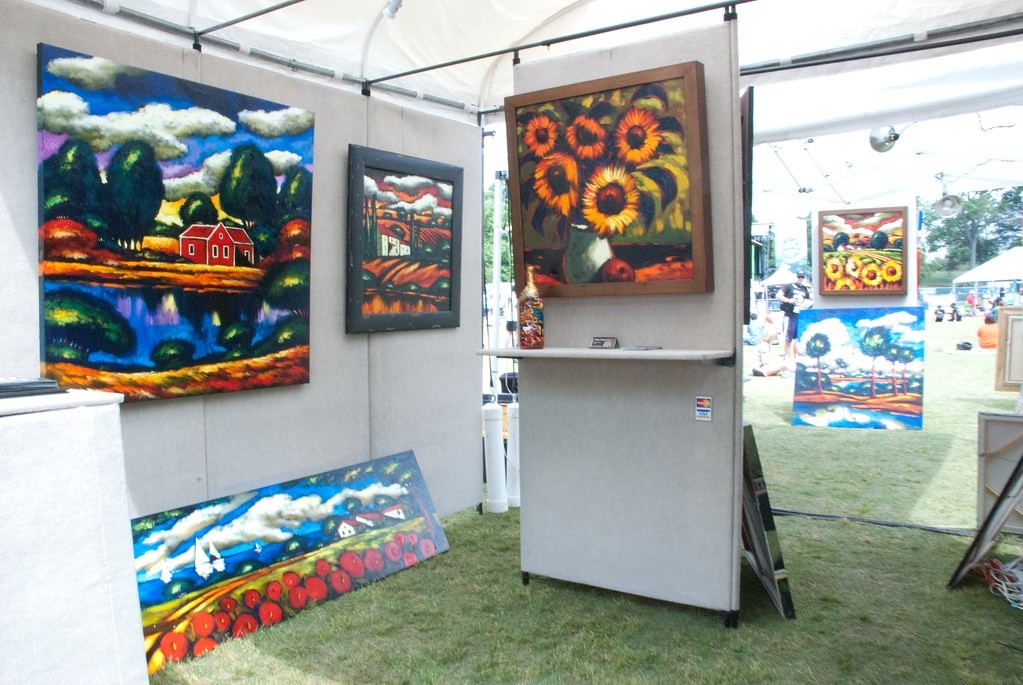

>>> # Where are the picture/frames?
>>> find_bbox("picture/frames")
[817,206,908,295]
[504,60,714,297]
[347,143,464,335]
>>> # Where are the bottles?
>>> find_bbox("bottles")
[520,265,545,349]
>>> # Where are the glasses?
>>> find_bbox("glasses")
[798,276,804,278]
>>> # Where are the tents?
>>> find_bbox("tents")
[758,264,807,313]
[953,245,1023,301]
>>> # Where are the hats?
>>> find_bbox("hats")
[798,271,804,276]
[949,303,956,306]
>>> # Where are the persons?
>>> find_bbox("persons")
[947,303,962,321]
[775,271,810,361]
[987,287,1007,321]
[977,315,999,349]
[744,313,779,345]
[966,290,976,308]
[931,305,945,322]
